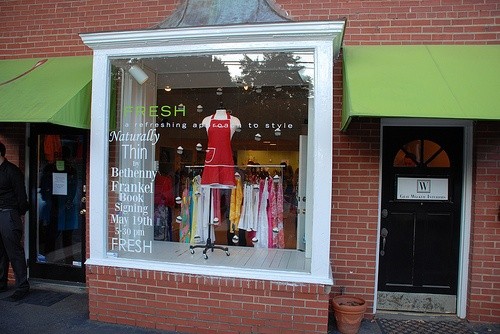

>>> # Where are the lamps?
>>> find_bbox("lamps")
[128,63,149,85]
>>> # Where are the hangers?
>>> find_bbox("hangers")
[185,165,280,181]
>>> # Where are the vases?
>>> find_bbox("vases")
[332,295,367,334]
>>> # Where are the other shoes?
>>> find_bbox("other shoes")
[0,286,7,293]
[15,280,29,296]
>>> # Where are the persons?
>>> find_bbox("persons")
[247,159,298,213]
[201,109,241,190]
[0,143,32,302]
[154,161,176,242]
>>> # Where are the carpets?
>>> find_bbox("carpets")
[1,289,73,307]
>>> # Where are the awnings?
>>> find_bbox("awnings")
[341,42,500,134]
[0,54,92,133]
